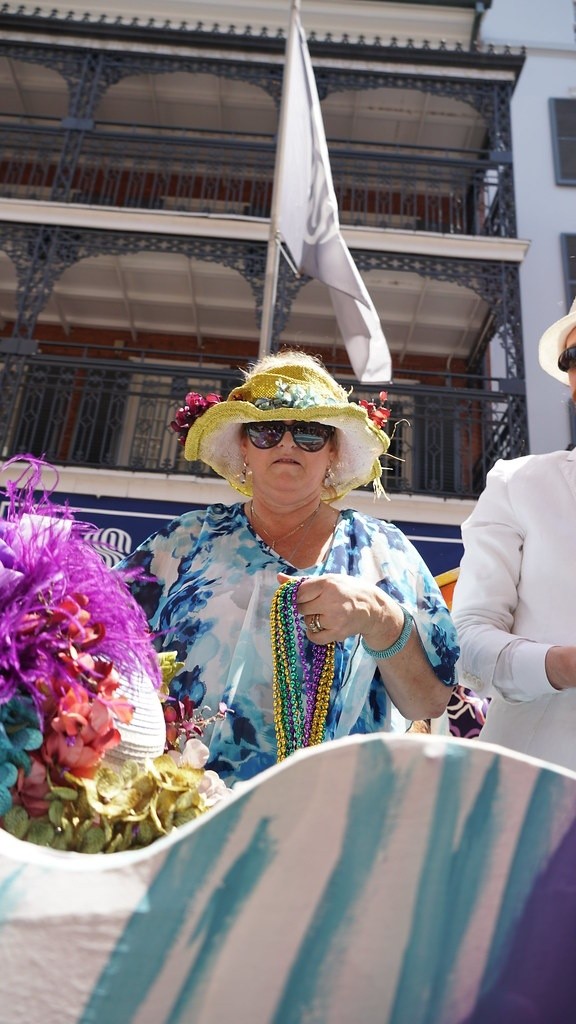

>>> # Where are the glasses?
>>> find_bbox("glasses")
[245,421,334,452]
[557,346,576,371]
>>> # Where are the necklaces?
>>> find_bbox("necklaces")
[250,500,321,563]
[271,577,336,764]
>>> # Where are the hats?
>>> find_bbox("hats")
[0,463,232,853]
[171,345,391,501]
[539,297,576,386]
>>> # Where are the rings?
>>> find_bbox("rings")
[310,615,318,632]
[316,615,324,631]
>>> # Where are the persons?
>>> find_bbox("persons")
[107,350,462,787]
[451,299,576,775]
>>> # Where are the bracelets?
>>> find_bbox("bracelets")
[361,607,413,658]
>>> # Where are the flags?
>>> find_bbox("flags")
[276,15,393,383]
